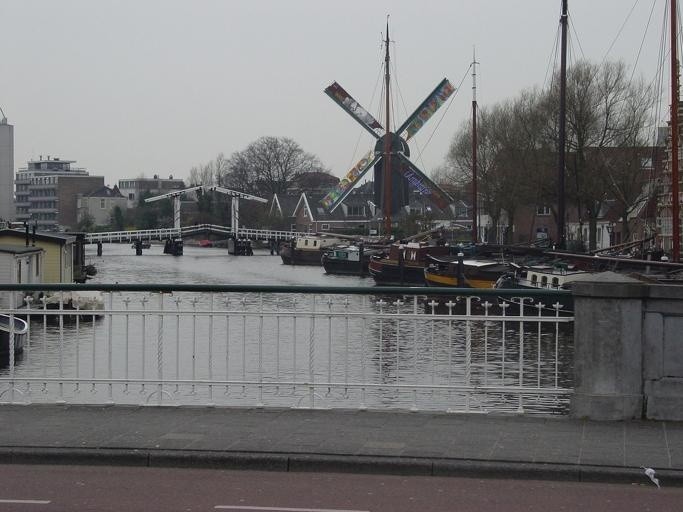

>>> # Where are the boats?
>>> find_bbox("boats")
[1,315,29,357]
[131,243,150,249]
